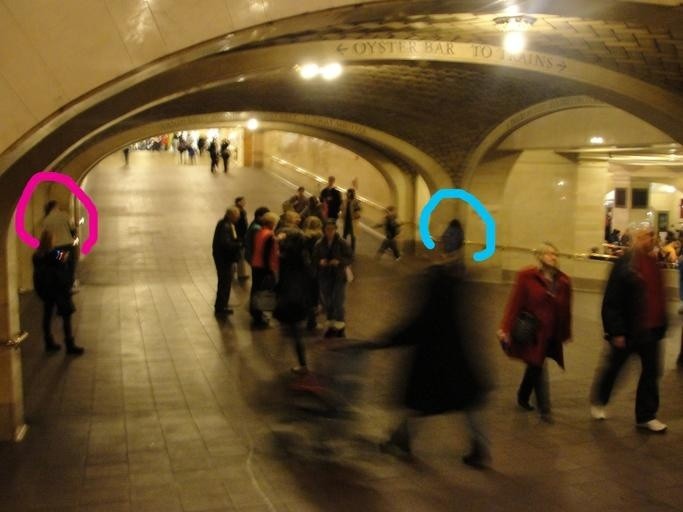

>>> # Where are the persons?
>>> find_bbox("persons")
[44,200,77,270]
[588,220,666,434]
[32,205,84,356]
[121,128,231,173]
[605,204,682,372]
[209,175,362,376]
[371,205,402,262]
[497,239,573,424]
[364,222,490,465]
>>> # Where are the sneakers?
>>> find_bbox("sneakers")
[589,401,607,419]
[637,417,669,433]
[213,301,347,340]
[290,366,310,380]
[517,394,554,424]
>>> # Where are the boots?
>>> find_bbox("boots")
[43,336,85,356]
[462,437,494,467]
[379,429,415,455]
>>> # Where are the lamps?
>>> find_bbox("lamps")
[491,1,536,53]
